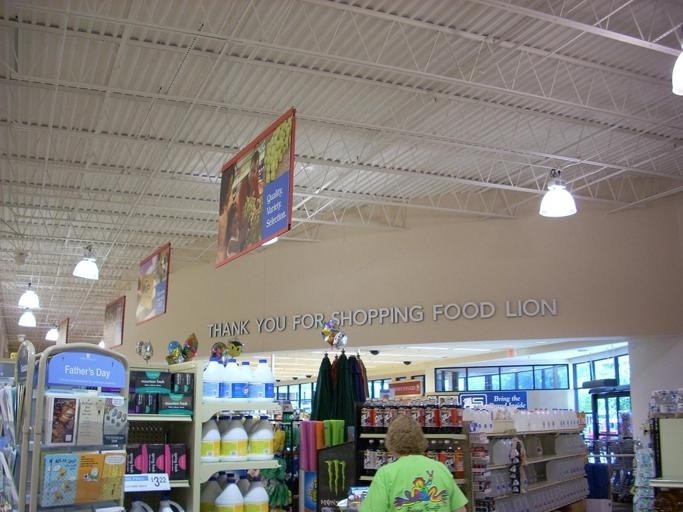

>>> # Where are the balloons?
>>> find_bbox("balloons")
[164,332,199,367]
[332,330,347,348]
[321,319,340,343]
[318,319,326,340]
[140,341,152,360]
[225,340,244,357]
[210,341,225,360]
[136,340,143,356]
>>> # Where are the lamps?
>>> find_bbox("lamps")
[537,165,577,217]
[17,286,59,340]
[71,247,100,280]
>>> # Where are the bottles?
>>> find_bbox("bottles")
[135,255,162,322]
[201,356,274,399]
[226,139,263,252]
[360,397,464,480]
[465,406,590,511]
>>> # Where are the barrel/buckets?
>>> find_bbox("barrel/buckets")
[201,471,270,512]
[201,414,276,462]
[128,495,187,512]
[461,404,589,512]
[584,463,609,500]
[202,355,275,398]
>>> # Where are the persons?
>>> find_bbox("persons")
[234,152,260,222]
[357,413,470,511]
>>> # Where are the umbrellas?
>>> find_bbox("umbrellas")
[311,353,332,419]
[331,348,355,441]
[348,353,367,403]
[355,354,370,397]
[330,353,338,380]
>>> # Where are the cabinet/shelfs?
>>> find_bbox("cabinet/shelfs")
[130,364,282,512]
[358,425,589,512]
